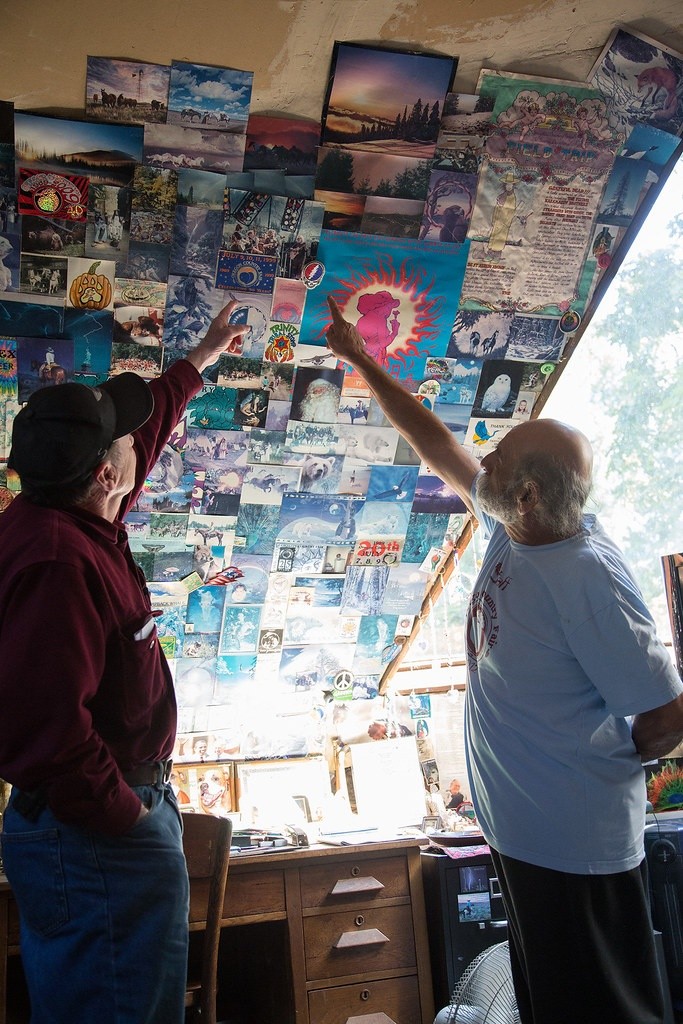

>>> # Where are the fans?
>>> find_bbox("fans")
[433,940,522,1024]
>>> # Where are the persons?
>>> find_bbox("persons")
[232,224,279,256]
[311,237,320,259]
[446,779,464,807]
[207,522,215,534]
[467,899,473,910]
[39,347,55,378]
[178,738,230,761]
[0,300,251,1024]
[91,209,123,247]
[515,400,529,414]
[326,295,683,1024]
[525,371,537,387]
[290,236,306,276]
[210,437,227,460]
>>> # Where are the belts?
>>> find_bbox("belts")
[120,760,175,786]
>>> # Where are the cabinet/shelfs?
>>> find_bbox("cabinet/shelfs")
[189,827,436,1024]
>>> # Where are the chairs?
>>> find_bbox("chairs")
[181,813,233,1024]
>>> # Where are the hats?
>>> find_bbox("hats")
[6,371,156,489]
